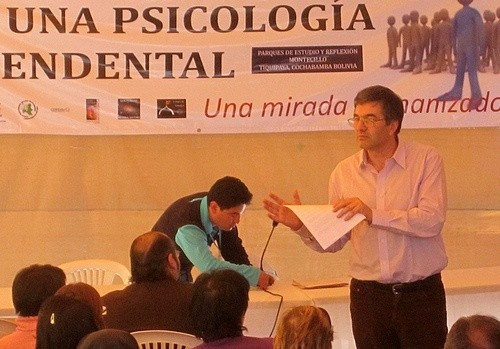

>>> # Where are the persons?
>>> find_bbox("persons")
[78,328,140,349]
[0,264,66,349]
[151,176,276,289]
[274,304,334,349]
[190,268,275,349]
[261,85,449,349]
[443,314,500,349]
[99,231,202,337]
[34,282,105,349]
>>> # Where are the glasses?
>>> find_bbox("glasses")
[348,118,390,129]
[100,306,106,317]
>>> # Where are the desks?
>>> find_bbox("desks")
[0,268,499,349]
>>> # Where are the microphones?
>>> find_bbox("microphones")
[258,220,279,287]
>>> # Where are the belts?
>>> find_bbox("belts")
[351,281,428,294]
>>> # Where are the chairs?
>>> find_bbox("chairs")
[131,331,202,349]
[58,259,131,284]
